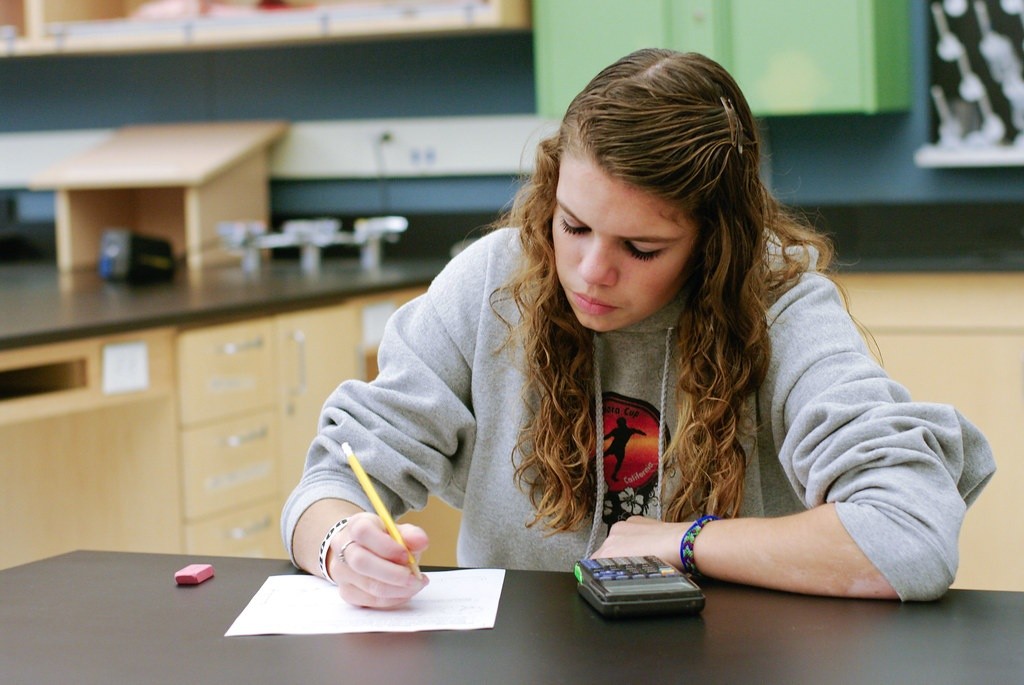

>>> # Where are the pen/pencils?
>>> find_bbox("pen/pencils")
[341,442,424,583]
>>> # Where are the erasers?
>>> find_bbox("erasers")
[174,563,214,584]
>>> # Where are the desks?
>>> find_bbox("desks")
[0,548,1024,685]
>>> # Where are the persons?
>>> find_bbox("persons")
[278,45,1000,615]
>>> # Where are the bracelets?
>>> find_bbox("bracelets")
[680,515,720,581]
[317,517,355,589]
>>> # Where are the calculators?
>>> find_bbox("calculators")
[573,554,707,620]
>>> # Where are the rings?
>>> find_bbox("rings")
[338,539,357,569]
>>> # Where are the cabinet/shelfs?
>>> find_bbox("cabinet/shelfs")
[533,0,915,118]
[0,327,178,577]
[828,272,1024,592]
[177,301,354,557]
[347,282,469,567]
